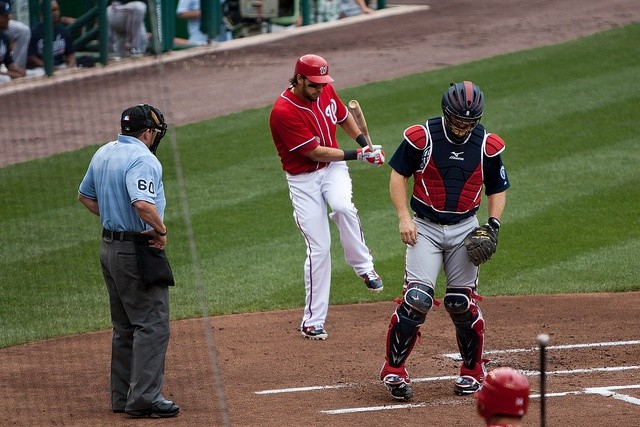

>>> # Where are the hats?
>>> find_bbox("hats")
[294,54,334,83]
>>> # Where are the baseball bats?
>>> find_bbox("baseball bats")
[348,100,373,152]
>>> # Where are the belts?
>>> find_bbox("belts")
[103,229,147,242]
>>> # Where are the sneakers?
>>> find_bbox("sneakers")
[359,271,383,293]
[301,326,328,340]
[381,367,413,400]
[455,364,487,395]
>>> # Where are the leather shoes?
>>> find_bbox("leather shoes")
[125,394,180,418]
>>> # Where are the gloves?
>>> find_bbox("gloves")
[356,145,385,167]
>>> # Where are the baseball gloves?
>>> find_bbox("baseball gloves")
[464,217,501,266]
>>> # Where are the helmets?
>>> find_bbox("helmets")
[477,368,529,419]
[122,104,167,155]
[442,81,484,144]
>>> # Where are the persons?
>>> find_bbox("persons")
[378,81,510,400]
[148,32,208,46]
[0,0,31,79]
[476,366,530,426]
[106,1,148,61]
[261,0,373,32]
[29,0,75,76]
[77,104,182,418]
[176,0,232,42]
[268,52,385,342]
